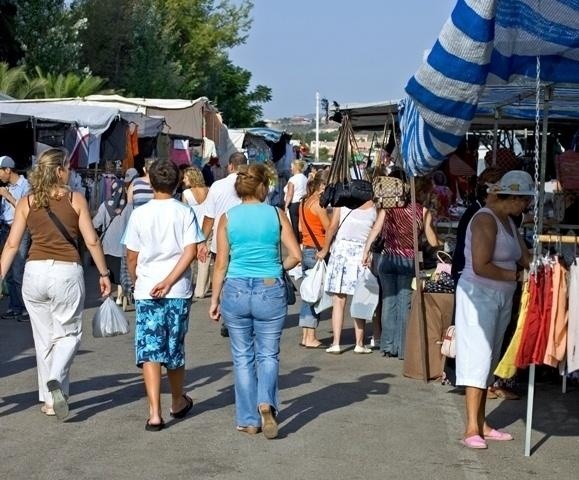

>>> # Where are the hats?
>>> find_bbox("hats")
[123,168,138,183]
[485,171,540,197]
[0,155,14,170]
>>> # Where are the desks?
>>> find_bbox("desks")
[399,286,458,384]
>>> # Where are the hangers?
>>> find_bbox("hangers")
[519,230,578,283]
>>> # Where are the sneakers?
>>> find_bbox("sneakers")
[486,387,520,401]
[2,308,30,322]
[236,422,259,436]
[41,379,69,420]
[258,402,279,439]
[364,337,380,349]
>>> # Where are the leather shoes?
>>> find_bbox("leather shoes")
[325,346,342,354]
[354,343,373,354]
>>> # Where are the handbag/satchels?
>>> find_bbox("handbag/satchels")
[283,273,298,305]
[418,233,457,294]
[440,324,457,358]
[319,168,407,209]
[370,236,386,254]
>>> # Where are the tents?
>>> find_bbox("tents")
[397,0,578,456]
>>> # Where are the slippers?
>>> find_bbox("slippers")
[171,394,193,417]
[299,341,330,349]
[145,418,164,430]
[462,428,512,449]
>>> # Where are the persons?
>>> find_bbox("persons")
[2,149,578,448]
